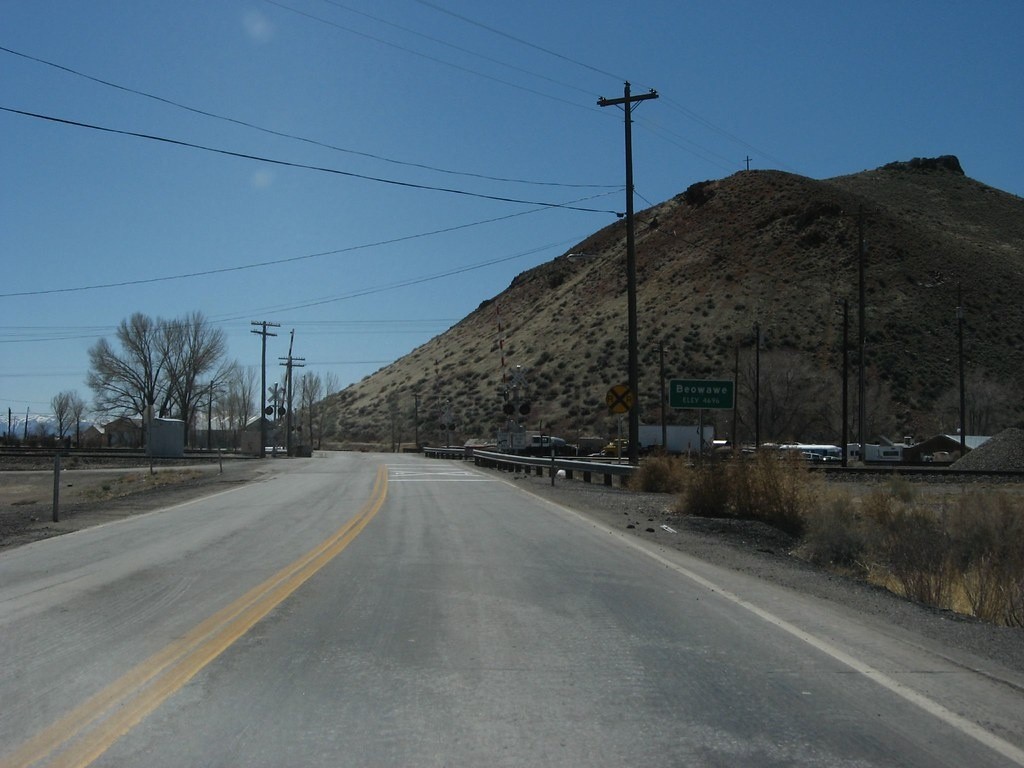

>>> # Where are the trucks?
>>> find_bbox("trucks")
[531,435,566,447]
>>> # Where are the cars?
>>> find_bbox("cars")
[605,439,629,457]
